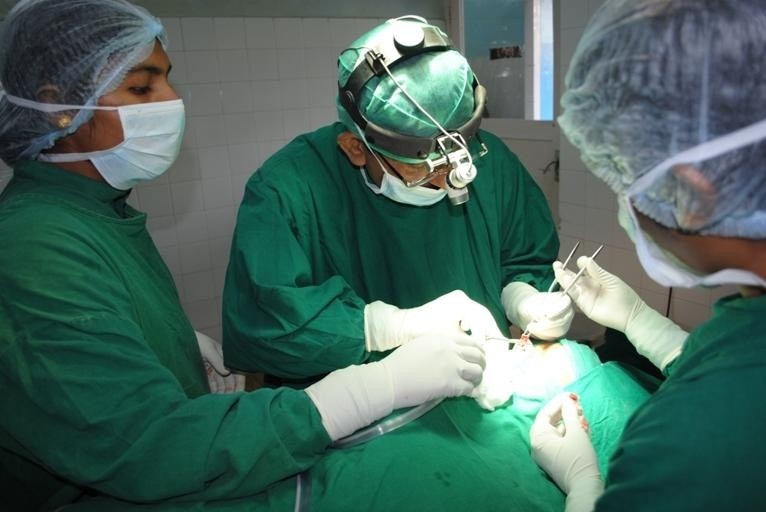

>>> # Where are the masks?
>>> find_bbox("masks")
[349,124,449,209]
[616,160,696,299]
[85,97,189,194]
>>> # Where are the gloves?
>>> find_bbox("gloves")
[552,251,690,373]
[190,326,246,397]
[359,285,510,357]
[500,282,576,342]
[303,330,490,444]
[528,391,607,512]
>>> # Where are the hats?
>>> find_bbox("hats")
[334,18,481,164]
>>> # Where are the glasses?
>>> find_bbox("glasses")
[352,118,454,190]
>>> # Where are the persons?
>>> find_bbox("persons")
[530,0,765,512]
[222,14,576,388]
[1,0,488,511]
